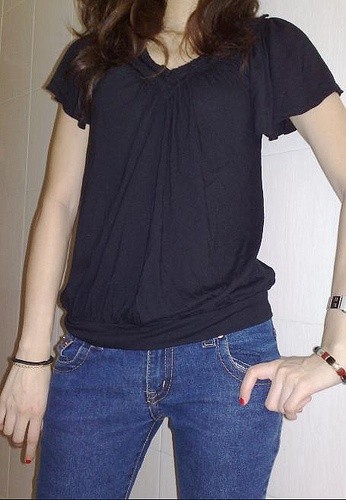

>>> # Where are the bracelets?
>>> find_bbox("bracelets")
[313,346,346,384]
[326,295,346,314]
[10,357,55,369]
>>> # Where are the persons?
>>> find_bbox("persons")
[0,0,346,500]
